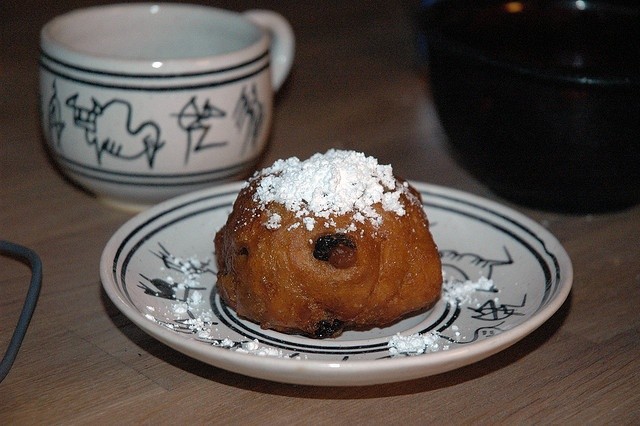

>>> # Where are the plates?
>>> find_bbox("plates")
[98,182,575,386]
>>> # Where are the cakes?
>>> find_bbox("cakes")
[212,148,442,340]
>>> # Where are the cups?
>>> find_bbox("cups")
[37,0,296,215]
